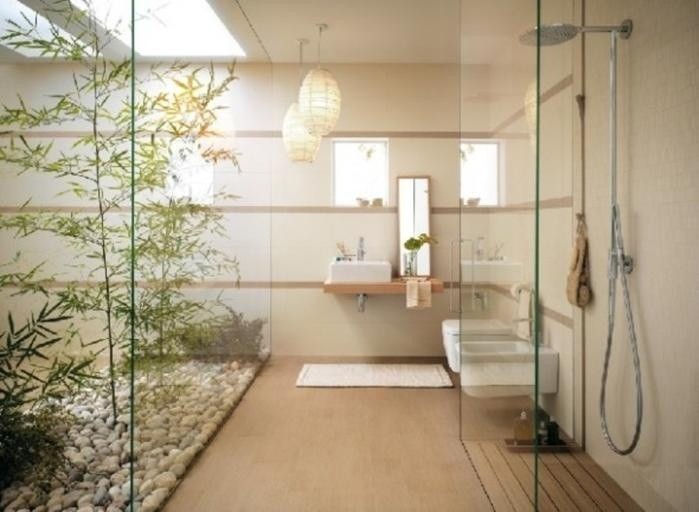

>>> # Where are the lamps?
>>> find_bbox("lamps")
[281,39,322,160]
[298,24,340,137]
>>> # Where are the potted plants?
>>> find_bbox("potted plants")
[402,233,438,278]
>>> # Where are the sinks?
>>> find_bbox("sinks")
[328,256,393,283]
[514,410,537,443]
[456,338,562,400]
[462,258,525,284]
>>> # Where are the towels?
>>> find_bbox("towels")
[566,236,592,307]
[406,277,432,308]
[516,291,534,341]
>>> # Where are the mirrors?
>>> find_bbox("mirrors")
[460,138,505,209]
[397,176,431,280]
[330,139,390,207]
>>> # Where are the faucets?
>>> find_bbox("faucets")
[532,332,546,348]
[476,234,487,260]
[356,234,368,258]
[509,280,534,316]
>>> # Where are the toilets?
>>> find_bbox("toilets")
[441,315,509,373]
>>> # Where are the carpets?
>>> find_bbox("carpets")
[295,363,454,388]
